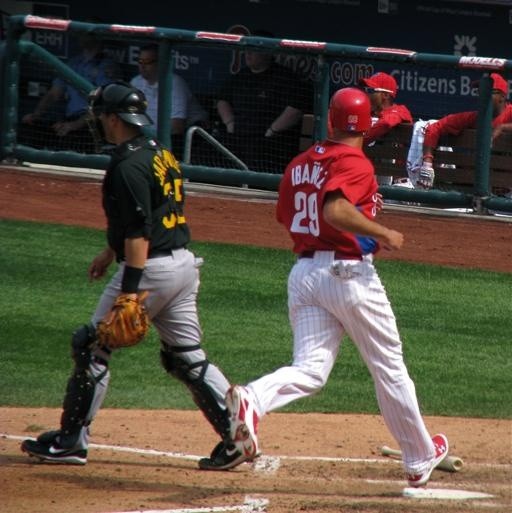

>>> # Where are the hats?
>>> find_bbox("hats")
[471,73,507,95]
[360,72,399,99]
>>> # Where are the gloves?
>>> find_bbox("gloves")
[417,162,434,191]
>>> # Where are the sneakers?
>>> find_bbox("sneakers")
[225,385,260,458]
[198,440,261,471]
[405,433,449,486]
[20,429,87,464]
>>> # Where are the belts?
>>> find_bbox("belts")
[116,246,187,264]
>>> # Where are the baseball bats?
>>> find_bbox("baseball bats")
[382,445,464,473]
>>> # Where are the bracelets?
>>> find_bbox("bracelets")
[122,265,142,291]
[267,125,281,137]
[422,154,435,159]
[227,118,234,126]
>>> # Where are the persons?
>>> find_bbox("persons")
[227,87,449,488]
[23,81,261,469]
[417,73,512,215]
[361,71,413,205]
[215,44,314,169]
[20,35,114,155]
[122,41,206,160]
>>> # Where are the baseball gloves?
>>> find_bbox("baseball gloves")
[95,291,150,349]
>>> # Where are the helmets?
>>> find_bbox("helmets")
[329,87,372,132]
[92,83,155,126]
